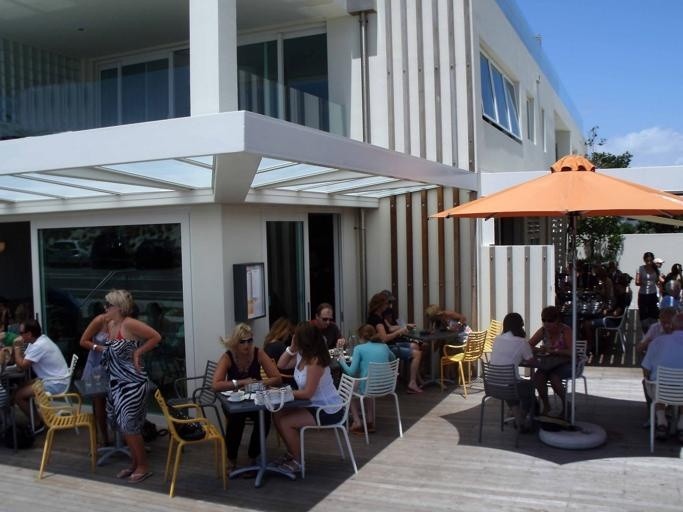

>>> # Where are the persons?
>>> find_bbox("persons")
[664,264,683,295]
[380,290,424,353]
[484,312,535,433]
[424,304,471,383]
[141,300,175,380]
[338,324,398,433]
[211,323,283,480]
[104,290,162,485]
[12,318,70,439]
[307,303,349,353]
[270,323,344,473]
[79,302,140,452]
[528,305,584,422]
[596,267,614,306]
[580,273,633,349]
[635,252,664,336]
[642,314,683,438]
[637,306,680,430]
[652,257,666,279]
[366,293,423,393]
[658,280,683,315]
[264,318,297,390]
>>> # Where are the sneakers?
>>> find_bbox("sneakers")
[28,423,45,433]
[657,421,668,444]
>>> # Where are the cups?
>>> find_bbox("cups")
[229,392,242,400]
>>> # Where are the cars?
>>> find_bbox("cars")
[47,241,82,267]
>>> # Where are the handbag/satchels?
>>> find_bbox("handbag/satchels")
[255,385,295,412]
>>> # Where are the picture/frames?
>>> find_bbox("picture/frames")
[234,262,267,322]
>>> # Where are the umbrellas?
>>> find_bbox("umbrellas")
[425,153,683,450]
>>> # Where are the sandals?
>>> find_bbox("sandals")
[276,452,291,464]
[282,459,309,472]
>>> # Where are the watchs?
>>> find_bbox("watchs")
[93,344,99,350]
[232,378,240,390]
[14,346,21,350]
[284,345,297,355]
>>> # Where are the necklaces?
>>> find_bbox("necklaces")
[234,352,252,374]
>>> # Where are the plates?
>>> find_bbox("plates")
[220,389,244,396]
[226,396,244,402]
[327,348,346,355]
[243,393,256,400]
[336,356,352,362]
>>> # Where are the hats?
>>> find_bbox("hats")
[654,257,664,264]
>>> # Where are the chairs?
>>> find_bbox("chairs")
[30,354,79,437]
[32,379,98,479]
[353,357,403,444]
[259,359,277,380]
[644,366,683,452]
[167,359,226,453]
[478,361,534,443]
[483,320,503,363]
[300,373,358,478]
[438,330,488,398]
[538,339,589,419]
[593,304,630,357]
[154,388,228,498]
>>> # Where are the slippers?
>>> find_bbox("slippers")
[408,387,426,394]
[367,422,375,434]
[225,463,234,474]
[117,469,132,478]
[351,427,365,437]
[128,471,153,483]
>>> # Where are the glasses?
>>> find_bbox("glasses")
[103,301,112,309]
[321,317,335,323]
[19,329,28,336]
[239,339,255,345]
